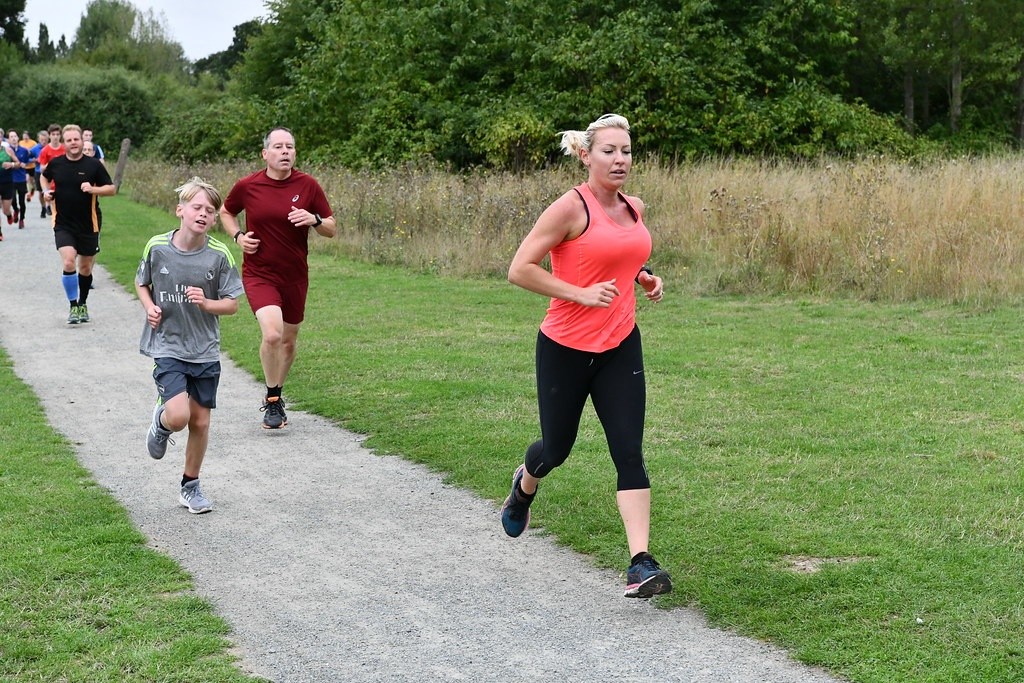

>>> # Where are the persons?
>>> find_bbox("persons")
[0,123,118,324]
[134,177,245,515]
[220,126,337,430]
[500,114,673,599]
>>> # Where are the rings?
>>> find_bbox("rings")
[300,216,303,222]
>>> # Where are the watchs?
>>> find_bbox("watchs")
[312,214,323,229]
[635,266,653,285]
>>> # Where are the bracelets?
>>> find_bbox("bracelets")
[232,231,245,244]
[12,161,15,168]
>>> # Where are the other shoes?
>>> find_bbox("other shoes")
[40,208,47,218]
[46,206,52,215]
[27,194,31,201]
[0,233,2,241]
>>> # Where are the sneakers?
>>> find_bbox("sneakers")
[624,553,673,598]
[146,403,176,459]
[19,220,24,229]
[178,479,212,513]
[259,396,288,428]
[7,214,12,224]
[67,306,80,324]
[501,463,539,538]
[78,304,91,322]
[13,208,19,222]
